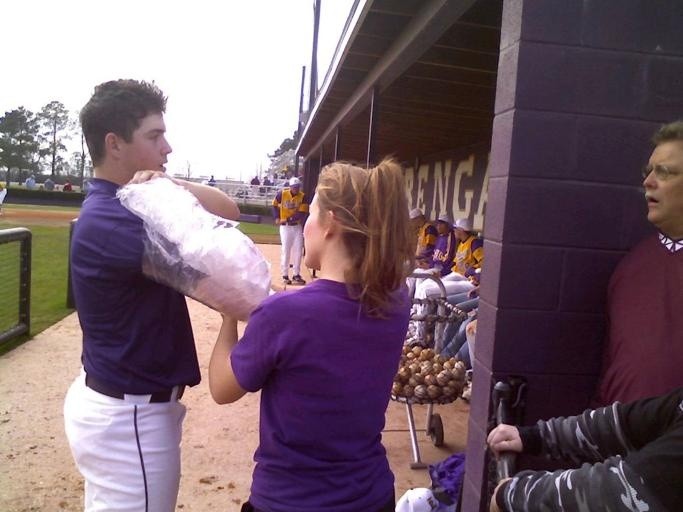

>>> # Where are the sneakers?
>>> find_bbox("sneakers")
[292,274,305,285]
[284,276,291,285]
[404,337,426,347]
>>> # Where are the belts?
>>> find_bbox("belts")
[281,222,297,225]
[85,374,186,404]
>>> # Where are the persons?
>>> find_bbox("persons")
[82,179,94,192]
[0,181,8,215]
[274,166,293,186]
[251,176,260,188]
[63,81,257,512]
[406,216,456,299]
[45,177,55,191]
[588,120,683,409]
[26,175,35,191]
[441,285,480,350]
[408,207,437,269]
[486,386,683,512]
[272,177,309,285]
[63,178,72,191]
[263,176,270,191]
[208,176,216,186]
[296,169,303,182]
[406,218,484,345]
[441,308,478,370]
[208,156,413,512]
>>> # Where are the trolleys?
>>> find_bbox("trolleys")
[380,272,468,469]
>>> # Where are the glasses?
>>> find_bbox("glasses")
[642,164,682,181]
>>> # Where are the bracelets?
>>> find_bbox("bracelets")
[283,284,288,292]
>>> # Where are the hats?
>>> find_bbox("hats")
[289,176,301,185]
[409,209,421,219]
[396,488,439,512]
[438,215,448,222]
[453,219,472,231]
